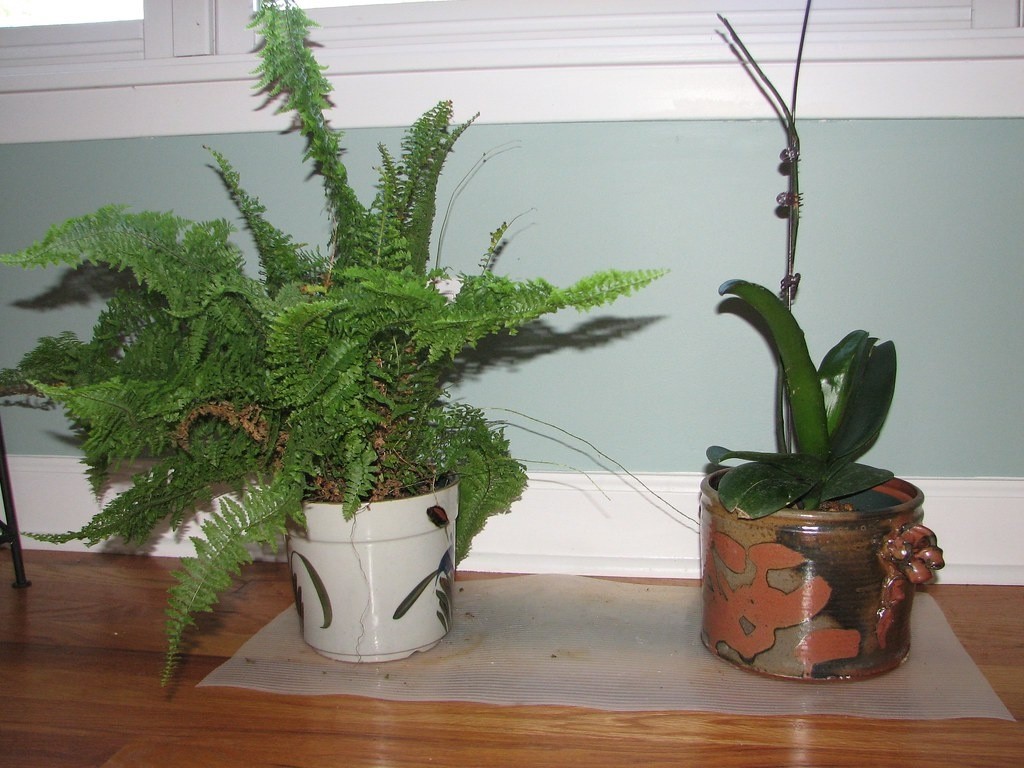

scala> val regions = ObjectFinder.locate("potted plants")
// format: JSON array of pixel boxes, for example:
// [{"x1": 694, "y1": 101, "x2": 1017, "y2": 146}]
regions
[
  {"x1": 0, "y1": 0, "x2": 669, "y2": 685},
  {"x1": 698, "y1": 0, "x2": 947, "y2": 682}
]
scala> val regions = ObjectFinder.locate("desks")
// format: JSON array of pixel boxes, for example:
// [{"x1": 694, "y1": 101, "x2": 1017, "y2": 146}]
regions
[{"x1": 0, "y1": 548, "x2": 1024, "y2": 768}]
